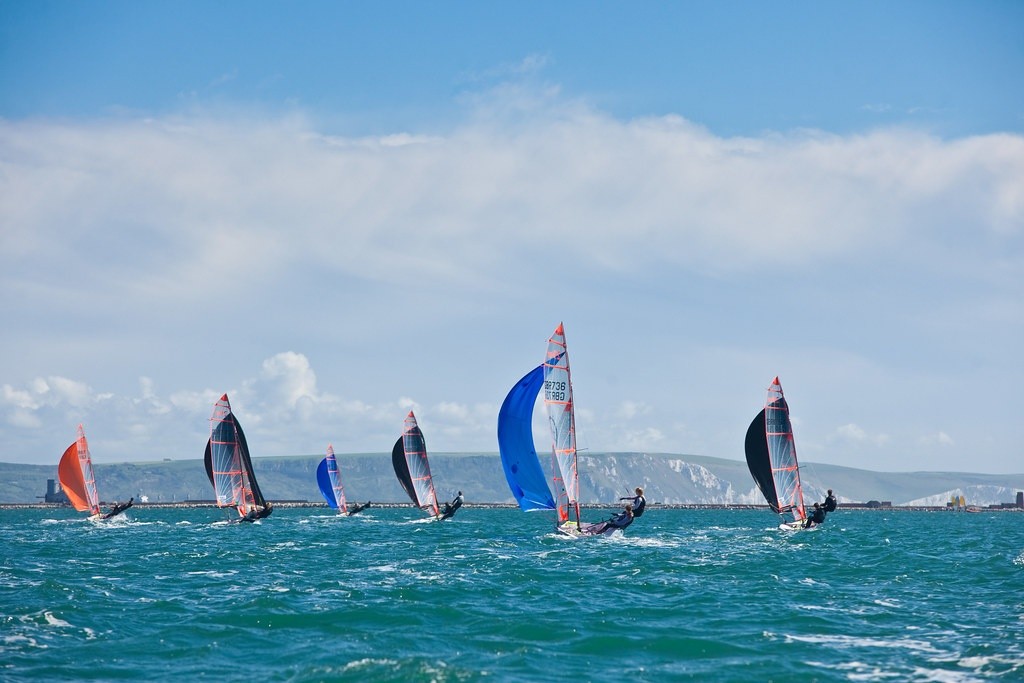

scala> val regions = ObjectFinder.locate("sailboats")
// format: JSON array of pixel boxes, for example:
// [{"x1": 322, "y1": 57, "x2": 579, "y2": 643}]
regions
[
  {"x1": 57, "y1": 436, "x2": 114, "y2": 523},
  {"x1": 391, "y1": 411, "x2": 451, "y2": 524},
  {"x1": 314, "y1": 444, "x2": 367, "y2": 518},
  {"x1": 744, "y1": 376, "x2": 819, "y2": 533},
  {"x1": 203, "y1": 392, "x2": 273, "y2": 526},
  {"x1": 495, "y1": 321, "x2": 625, "y2": 543}
]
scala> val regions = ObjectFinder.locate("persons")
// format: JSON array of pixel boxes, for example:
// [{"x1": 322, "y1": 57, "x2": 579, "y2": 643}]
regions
[
  {"x1": 441, "y1": 502, "x2": 454, "y2": 520},
  {"x1": 239, "y1": 506, "x2": 257, "y2": 523},
  {"x1": 451, "y1": 491, "x2": 464, "y2": 512},
  {"x1": 103, "y1": 498, "x2": 134, "y2": 519},
  {"x1": 257, "y1": 502, "x2": 273, "y2": 519},
  {"x1": 618, "y1": 488, "x2": 646, "y2": 521},
  {"x1": 596, "y1": 505, "x2": 634, "y2": 535},
  {"x1": 349, "y1": 501, "x2": 370, "y2": 516},
  {"x1": 806, "y1": 502, "x2": 825, "y2": 529},
  {"x1": 821, "y1": 490, "x2": 837, "y2": 515}
]
